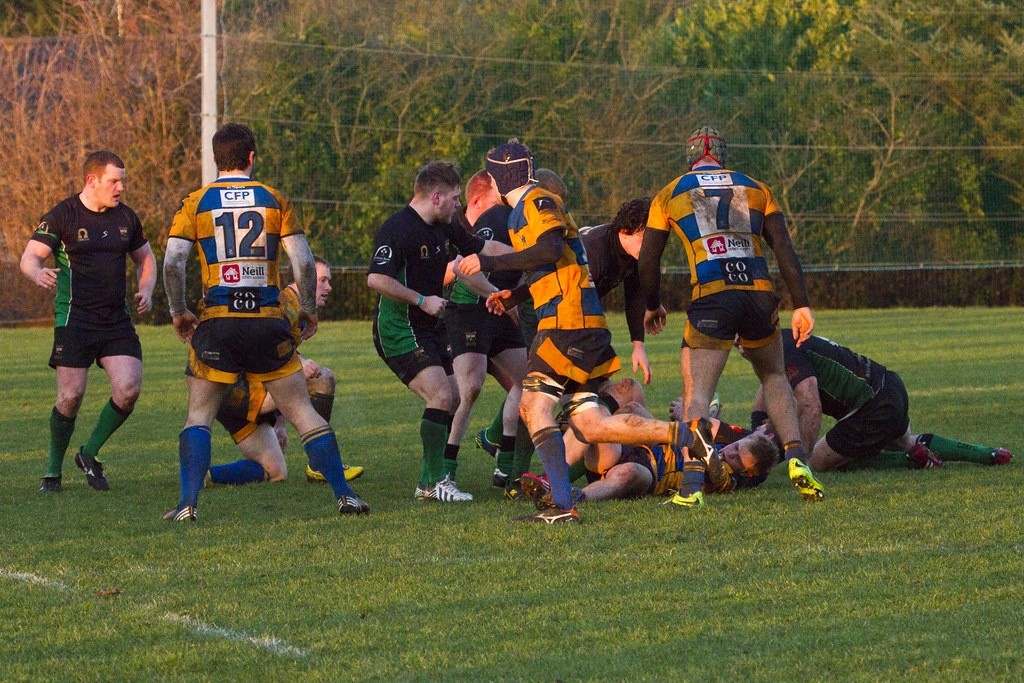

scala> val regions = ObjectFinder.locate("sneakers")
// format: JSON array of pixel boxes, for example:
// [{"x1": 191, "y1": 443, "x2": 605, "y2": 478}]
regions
[
  {"x1": 425, "y1": 473, "x2": 473, "y2": 501},
  {"x1": 339, "y1": 496, "x2": 369, "y2": 513},
  {"x1": 75, "y1": 453, "x2": 110, "y2": 491},
  {"x1": 520, "y1": 472, "x2": 551, "y2": 500},
  {"x1": 40, "y1": 476, "x2": 62, "y2": 492},
  {"x1": 518, "y1": 508, "x2": 582, "y2": 525},
  {"x1": 306, "y1": 465, "x2": 363, "y2": 483},
  {"x1": 503, "y1": 478, "x2": 533, "y2": 502},
  {"x1": 492, "y1": 468, "x2": 509, "y2": 489},
  {"x1": 906, "y1": 444, "x2": 941, "y2": 469},
  {"x1": 789, "y1": 458, "x2": 824, "y2": 501},
  {"x1": 662, "y1": 491, "x2": 704, "y2": 507},
  {"x1": 475, "y1": 428, "x2": 500, "y2": 458},
  {"x1": 163, "y1": 506, "x2": 197, "y2": 521},
  {"x1": 685, "y1": 416, "x2": 724, "y2": 483},
  {"x1": 993, "y1": 447, "x2": 1010, "y2": 465},
  {"x1": 414, "y1": 486, "x2": 424, "y2": 499},
  {"x1": 535, "y1": 487, "x2": 586, "y2": 511}
]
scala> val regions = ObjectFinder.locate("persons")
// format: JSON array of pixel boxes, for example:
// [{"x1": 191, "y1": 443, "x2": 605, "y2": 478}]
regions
[
  {"x1": 441, "y1": 166, "x2": 569, "y2": 489},
  {"x1": 202, "y1": 255, "x2": 363, "y2": 490},
  {"x1": 734, "y1": 325, "x2": 1015, "y2": 470},
  {"x1": 367, "y1": 162, "x2": 515, "y2": 501},
  {"x1": 459, "y1": 142, "x2": 621, "y2": 524},
  {"x1": 163, "y1": 123, "x2": 372, "y2": 521},
  {"x1": 578, "y1": 196, "x2": 654, "y2": 387},
  {"x1": 636, "y1": 125, "x2": 825, "y2": 509},
  {"x1": 522, "y1": 379, "x2": 784, "y2": 511},
  {"x1": 20, "y1": 150, "x2": 157, "y2": 494}
]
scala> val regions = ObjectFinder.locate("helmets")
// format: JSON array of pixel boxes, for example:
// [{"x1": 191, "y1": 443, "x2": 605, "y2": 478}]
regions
[
  {"x1": 686, "y1": 128, "x2": 725, "y2": 166},
  {"x1": 485, "y1": 143, "x2": 533, "y2": 195}
]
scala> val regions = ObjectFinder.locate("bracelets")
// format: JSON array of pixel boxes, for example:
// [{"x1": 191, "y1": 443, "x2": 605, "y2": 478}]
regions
[{"x1": 416, "y1": 294, "x2": 424, "y2": 306}]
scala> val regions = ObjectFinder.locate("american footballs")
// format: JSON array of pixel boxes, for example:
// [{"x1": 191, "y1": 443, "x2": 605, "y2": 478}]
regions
[{"x1": 708, "y1": 391, "x2": 720, "y2": 418}]
